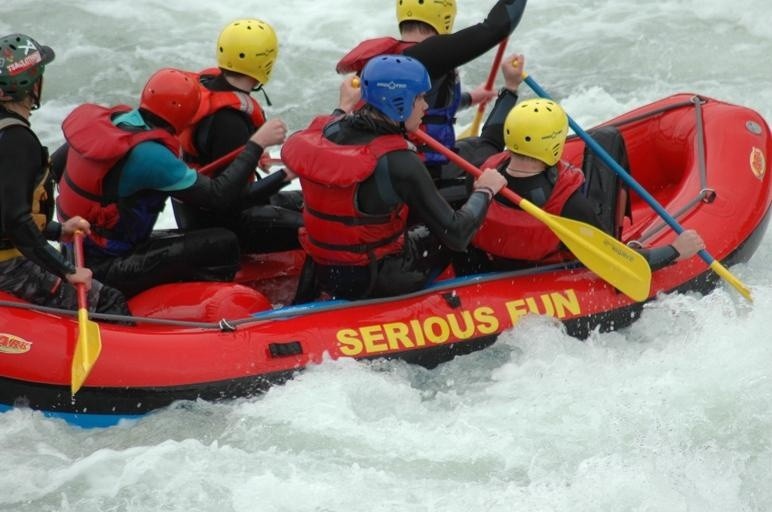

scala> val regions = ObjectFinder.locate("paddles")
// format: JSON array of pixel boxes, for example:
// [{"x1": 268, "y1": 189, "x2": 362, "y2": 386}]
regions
[
  {"x1": 519, "y1": 68, "x2": 754, "y2": 317},
  {"x1": 69, "y1": 231, "x2": 103, "y2": 397},
  {"x1": 415, "y1": 128, "x2": 651, "y2": 303}
]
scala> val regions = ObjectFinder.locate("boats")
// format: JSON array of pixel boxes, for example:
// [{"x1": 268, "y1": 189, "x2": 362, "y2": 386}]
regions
[{"x1": 0, "y1": 94, "x2": 768, "y2": 430}]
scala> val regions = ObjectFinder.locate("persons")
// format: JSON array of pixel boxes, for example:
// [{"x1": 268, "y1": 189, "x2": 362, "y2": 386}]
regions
[
  {"x1": 280, "y1": 55, "x2": 508, "y2": 300},
  {"x1": 1, "y1": 33, "x2": 138, "y2": 326},
  {"x1": 49, "y1": 68, "x2": 287, "y2": 297},
  {"x1": 455, "y1": 54, "x2": 707, "y2": 277},
  {"x1": 336, "y1": 0, "x2": 527, "y2": 181},
  {"x1": 173, "y1": 19, "x2": 304, "y2": 254}
]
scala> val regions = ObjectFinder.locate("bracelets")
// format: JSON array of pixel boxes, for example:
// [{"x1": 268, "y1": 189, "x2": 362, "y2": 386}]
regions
[{"x1": 477, "y1": 187, "x2": 494, "y2": 199}]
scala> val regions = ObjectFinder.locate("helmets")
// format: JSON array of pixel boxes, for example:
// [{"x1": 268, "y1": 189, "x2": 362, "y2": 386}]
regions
[
  {"x1": 217, "y1": 19, "x2": 279, "y2": 85},
  {"x1": 359, "y1": 54, "x2": 432, "y2": 123},
  {"x1": 503, "y1": 99, "x2": 569, "y2": 167},
  {"x1": 396, "y1": 0, "x2": 456, "y2": 35},
  {"x1": 0, "y1": 33, "x2": 56, "y2": 93},
  {"x1": 140, "y1": 68, "x2": 202, "y2": 138}
]
